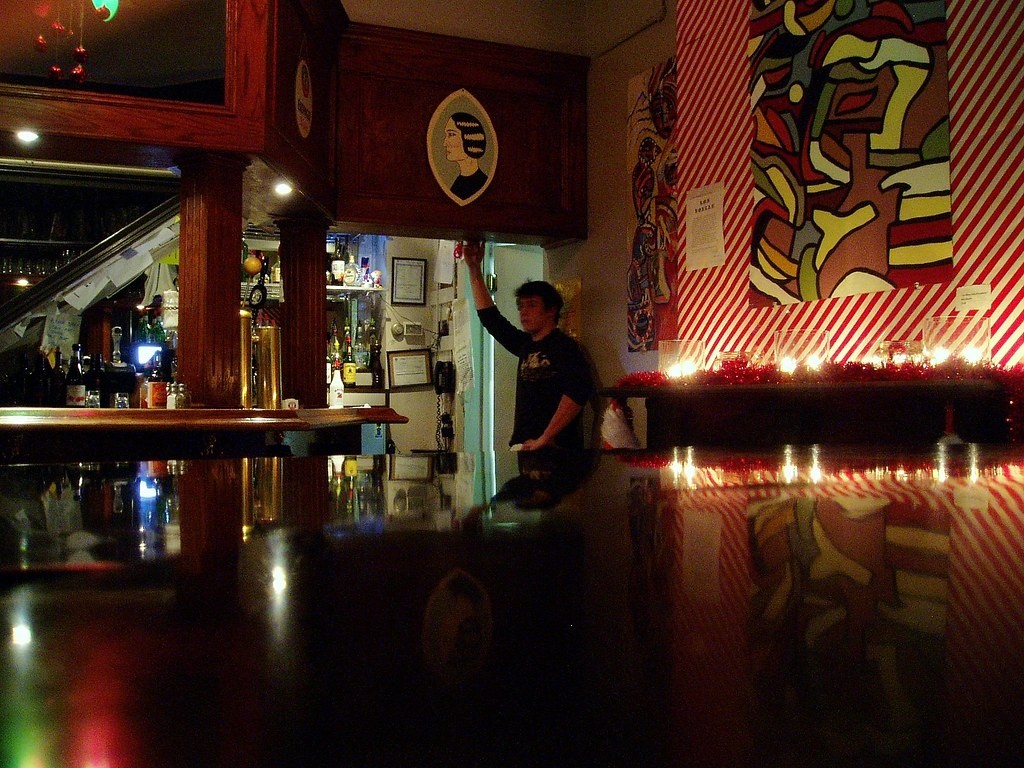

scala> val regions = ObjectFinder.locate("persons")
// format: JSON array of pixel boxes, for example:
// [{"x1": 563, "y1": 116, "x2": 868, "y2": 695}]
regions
[{"x1": 464, "y1": 240, "x2": 599, "y2": 513}]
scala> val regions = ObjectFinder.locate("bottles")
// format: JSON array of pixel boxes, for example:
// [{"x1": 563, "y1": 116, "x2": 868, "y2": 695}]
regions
[
  {"x1": 329, "y1": 470, "x2": 376, "y2": 524},
  {"x1": 167, "y1": 384, "x2": 177, "y2": 409},
  {"x1": 53, "y1": 352, "x2": 66, "y2": 407},
  {"x1": 326, "y1": 340, "x2": 333, "y2": 384},
  {"x1": 66, "y1": 344, "x2": 85, "y2": 407},
  {"x1": 20, "y1": 353, "x2": 51, "y2": 407},
  {"x1": 85, "y1": 354, "x2": 103, "y2": 408},
  {"x1": 330, "y1": 369, "x2": 345, "y2": 409},
  {"x1": 343, "y1": 343, "x2": 356, "y2": 388},
  {"x1": 175, "y1": 384, "x2": 191, "y2": 410}
]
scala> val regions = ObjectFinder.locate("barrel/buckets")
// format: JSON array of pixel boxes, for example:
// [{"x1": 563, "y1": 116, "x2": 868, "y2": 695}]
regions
[{"x1": 147, "y1": 382, "x2": 167, "y2": 409}]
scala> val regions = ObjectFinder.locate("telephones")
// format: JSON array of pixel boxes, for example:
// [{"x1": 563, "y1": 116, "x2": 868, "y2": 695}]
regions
[{"x1": 434, "y1": 361, "x2": 453, "y2": 395}]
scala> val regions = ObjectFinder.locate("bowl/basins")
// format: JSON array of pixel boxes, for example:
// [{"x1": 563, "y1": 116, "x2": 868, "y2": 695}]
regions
[
  {"x1": 657, "y1": 340, "x2": 708, "y2": 380},
  {"x1": 923, "y1": 316, "x2": 992, "y2": 364},
  {"x1": 771, "y1": 329, "x2": 831, "y2": 372}
]
